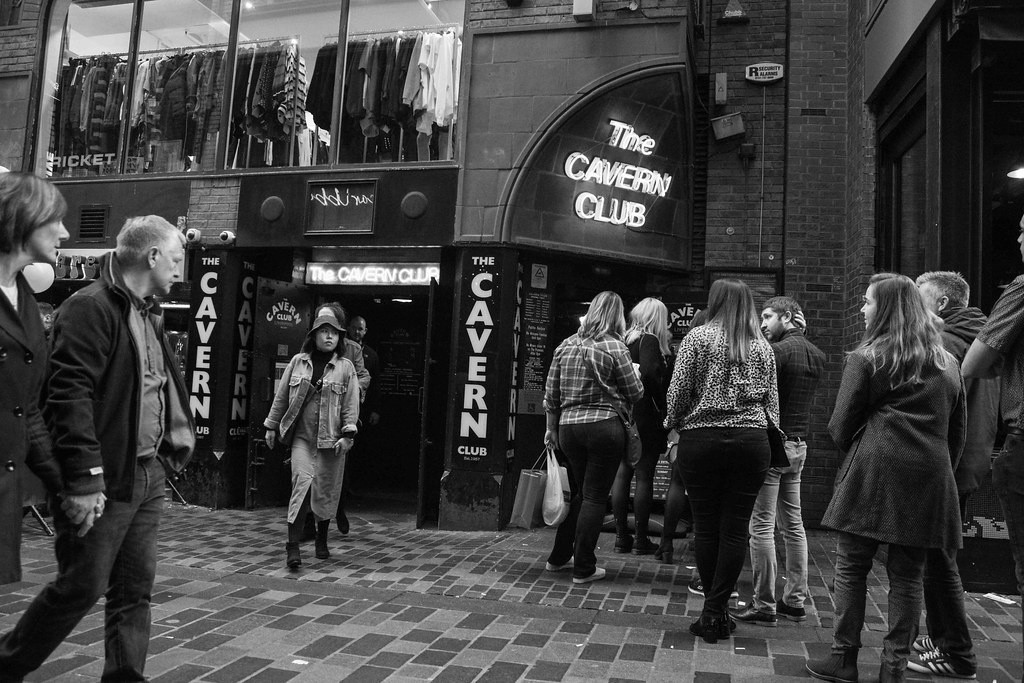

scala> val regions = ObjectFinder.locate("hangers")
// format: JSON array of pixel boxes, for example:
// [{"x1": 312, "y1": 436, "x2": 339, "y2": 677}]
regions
[{"x1": 63, "y1": 22, "x2": 463, "y2": 69}]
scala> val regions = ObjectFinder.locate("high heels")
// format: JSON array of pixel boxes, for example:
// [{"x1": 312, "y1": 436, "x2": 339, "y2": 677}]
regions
[
  {"x1": 688, "y1": 615, "x2": 719, "y2": 643},
  {"x1": 719, "y1": 610, "x2": 732, "y2": 639},
  {"x1": 654, "y1": 544, "x2": 674, "y2": 563}
]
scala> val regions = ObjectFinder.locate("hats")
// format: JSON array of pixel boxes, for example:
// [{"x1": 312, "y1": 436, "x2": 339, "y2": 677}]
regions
[{"x1": 306, "y1": 307, "x2": 347, "y2": 339}]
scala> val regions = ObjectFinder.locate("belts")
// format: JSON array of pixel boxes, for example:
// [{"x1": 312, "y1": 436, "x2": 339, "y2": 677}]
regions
[{"x1": 785, "y1": 436, "x2": 807, "y2": 442}]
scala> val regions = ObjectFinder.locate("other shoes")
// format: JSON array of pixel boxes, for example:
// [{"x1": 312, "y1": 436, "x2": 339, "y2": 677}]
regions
[
  {"x1": 879, "y1": 665, "x2": 907, "y2": 683},
  {"x1": 688, "y1": 577, "x2": 739, "y2": 598},
  {"x1": 806, "y1": 648, "x2": 858, "y2": 683},
  {"x1": 281, "y1": 505, "x2": 350, "y2": 568},
  {"x1": 544, "y1": 555, "x2": 575, "y2": 571},
  {"x1": 573, "y1": 566, "x2": 606, "y2": 583}
]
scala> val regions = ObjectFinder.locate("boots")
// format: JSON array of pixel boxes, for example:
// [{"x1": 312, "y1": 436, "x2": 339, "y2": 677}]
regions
[
  {"x1": 612, "y1": 511, "x2": 635, "y2": 552},
  {"x1": 632, "y1": 514, "x2": 659, "y2": 554}
]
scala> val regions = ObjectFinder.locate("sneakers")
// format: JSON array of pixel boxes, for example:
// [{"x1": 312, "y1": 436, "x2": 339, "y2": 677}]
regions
[
  {"x1": 912, "y1": 635, "x2": 936, "y2": 651},
  {"x1": 907, "y1": 647, "x2": 977, "y2": 679}
]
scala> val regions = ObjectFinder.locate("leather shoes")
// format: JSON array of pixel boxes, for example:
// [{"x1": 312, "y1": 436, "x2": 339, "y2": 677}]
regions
[
  {"x1": 729, "y1": 603, "x2": 778, "y2": 627},
  {"x1": 776, "y1": 598, "x2": 807, "y2": 622}
]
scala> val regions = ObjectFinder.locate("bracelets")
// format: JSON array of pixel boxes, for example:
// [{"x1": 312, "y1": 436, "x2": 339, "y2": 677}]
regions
[{"x1": 547, "y1": 423, "x2": 558, "y2": 431}]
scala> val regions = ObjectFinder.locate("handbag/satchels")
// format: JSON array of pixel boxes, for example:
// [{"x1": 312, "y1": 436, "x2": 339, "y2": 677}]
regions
[
  {"x1": 509, "y1": 446, "x2": 546, "y2": 529},
  {"x1": 542, "y1": 444, "x2": 570, "y2": 527},
  {"x1": 624, "y1": 419, "x2": 643, "y2": 468},
  {"x1": 764, "y1": 425, "x2": 790, "y2": 469},
  {"x1": 643, "y1": 407, "x2": 671, "y2": 432}
]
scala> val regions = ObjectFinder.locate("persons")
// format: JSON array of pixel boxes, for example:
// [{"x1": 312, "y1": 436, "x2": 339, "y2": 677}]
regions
[
  {"x1": 727, "y1": 294, "x2": 826, "y2": 628},
  {"x1": 1, "y1": 171, "x2": 107, "y2": 584},
  {"x1": 665, "y1": 278, "x2": 791, "y2": 643},
  {"x1": 37, "y1": 300, "x2": 54, "y2": 336},
  {"x1": 612, "y1": 298, "x2": 711, "y2": 563},
  {"x1": 806, "y1": 272, "x2": 967, "y2": 682},
  {"x1": 263, "y1": 303, "x2": 380, "y2": 567},
  {"x1": 0, "y1": 215, "x2": 199, "y2": 683},
  {"x1": 907, "y1": 212, "x2": 1024, "y2": 678},
  {"x1": 542, "y1": 291, "x2": 645, "y2": 583}
]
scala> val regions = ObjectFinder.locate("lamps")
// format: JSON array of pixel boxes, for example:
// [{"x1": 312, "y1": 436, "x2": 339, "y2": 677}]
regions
[{"x1": 1007, "y1": 158, "x2": 1024, "y2": 180}]
